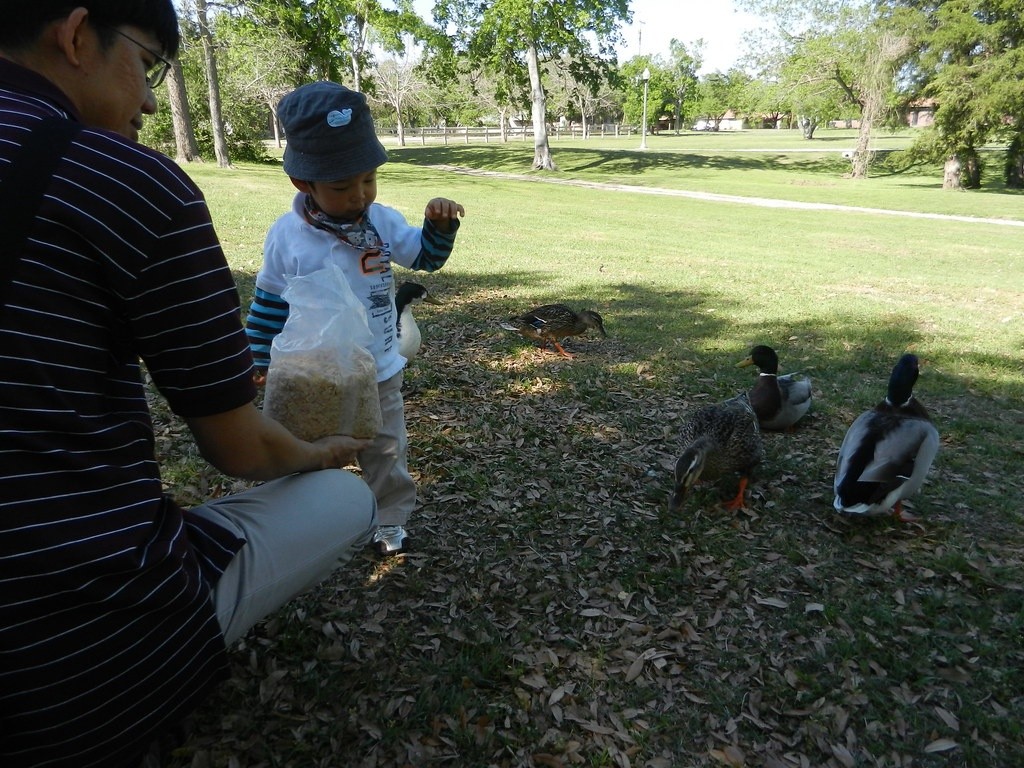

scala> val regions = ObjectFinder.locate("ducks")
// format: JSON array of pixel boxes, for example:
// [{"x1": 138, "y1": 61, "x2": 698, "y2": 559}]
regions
[
  {"x1": 733, "y1": 345, "x2": 813, "y2": 435},
  {"x1": 498, "y1": 304, "x2": 607, "y2": 359},
  {"x1": 834, "y1": 354, "x2": 940, "y2": 526},
  {"x1": 395, "y1": 281, "x2": 443, "y2": 365},
  {"x1": 669, "y1": 391, "x2": 763, "y2": 514}
]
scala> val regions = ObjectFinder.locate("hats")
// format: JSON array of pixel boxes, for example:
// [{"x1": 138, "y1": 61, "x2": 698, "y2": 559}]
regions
[{"x1": 276, "y1": 79, "x2": 388, "y2": 183}]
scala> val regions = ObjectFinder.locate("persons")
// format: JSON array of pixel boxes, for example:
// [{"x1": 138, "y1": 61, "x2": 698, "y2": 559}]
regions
[
  {"x1": 242, "y1": 80, "x2": 466, "y2": 559},
  {"x1": 0, "y1": 0, "x2": 380, "y2": 768}
]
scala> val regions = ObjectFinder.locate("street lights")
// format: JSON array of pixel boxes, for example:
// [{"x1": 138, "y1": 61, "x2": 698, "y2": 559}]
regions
[{"x1": 640, "y1": 67, "x2": 651, "y2": 148}]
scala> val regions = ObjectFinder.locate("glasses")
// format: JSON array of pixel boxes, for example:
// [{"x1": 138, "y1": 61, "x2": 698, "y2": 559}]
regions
[{"x1": 101, "y1": 23, "x2": 173, "y2": 91}]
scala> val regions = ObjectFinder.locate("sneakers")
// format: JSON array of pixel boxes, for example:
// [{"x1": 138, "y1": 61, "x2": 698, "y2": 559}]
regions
[{"x1": 373, "y1": 524, "x2": 411, "y2": 556}]
